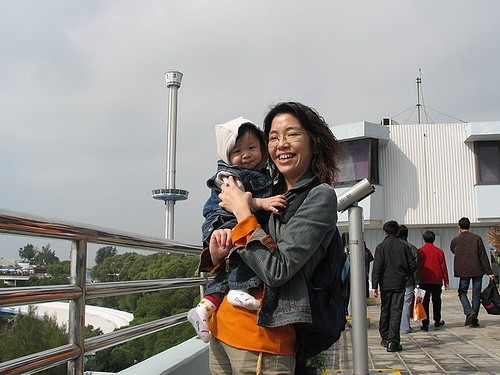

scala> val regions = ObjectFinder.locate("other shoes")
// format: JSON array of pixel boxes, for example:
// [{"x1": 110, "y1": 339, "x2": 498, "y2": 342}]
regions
[
  {"x1": 472, "y1": 318, "x2": 479, "y2": 327},
  {"x1": 421, "y1": 326, "x2": 428, "y2": 331},
  {"x1": 381, "y1": 340, "x2": 388, "y2": 347},
  {"x1": 387, "y1": 342, "x2": 402, "y2": 352},
  {"x1": 434, "y1": 321, "x2": 444, "y2": 327},
  {"x1": 465, "y1": 312, "x2": 476, "y2": 326},
  {"x1": 401, "y1": 327, "x2": 416, "y2": 334}
]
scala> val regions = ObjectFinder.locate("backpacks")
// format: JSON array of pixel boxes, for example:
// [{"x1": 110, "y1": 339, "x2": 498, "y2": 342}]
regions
[{"x1": 283, "y1": 179, "x2": 349, "y2": 363}]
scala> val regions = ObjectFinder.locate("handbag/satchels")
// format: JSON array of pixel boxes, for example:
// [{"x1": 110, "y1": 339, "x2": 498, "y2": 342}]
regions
[
  {"x1": 414, "y1": 288, "x2": 428, "y2": 322},
  {"x1": 479, "y1": 280, "x2": 500, "y2": 315}
]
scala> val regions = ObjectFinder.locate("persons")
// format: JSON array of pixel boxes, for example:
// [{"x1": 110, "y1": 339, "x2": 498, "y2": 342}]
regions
[
  {"x1": 450, "y1": 217, "x2": 496, "y2": 328},
  {"x1": 187, "y1": 117, "x2": 288, "y2": 344},
  {"x1": 372, "y1": 220, "x2": 416, "y2": 352},
  {"x1": 418, "y1": 231, "x2": 449, "y2": 331},
  {"x1": 196, "y1": 101, "x2": 342, "y2": 375},
  {"x1": 340, "y1": 237, "x2": 350, "y2": 330},
  {"x1": 397, "y1": 225, "x2": 419, "y2": 334},
  {"x1": 364, "y1": 241, "x2": 373, "y2": 299}
]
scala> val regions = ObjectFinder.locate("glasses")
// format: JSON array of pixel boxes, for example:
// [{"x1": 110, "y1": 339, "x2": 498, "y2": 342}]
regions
[{"x1": 265, "y1": 132, "x2": 308, "y2": 146}]
